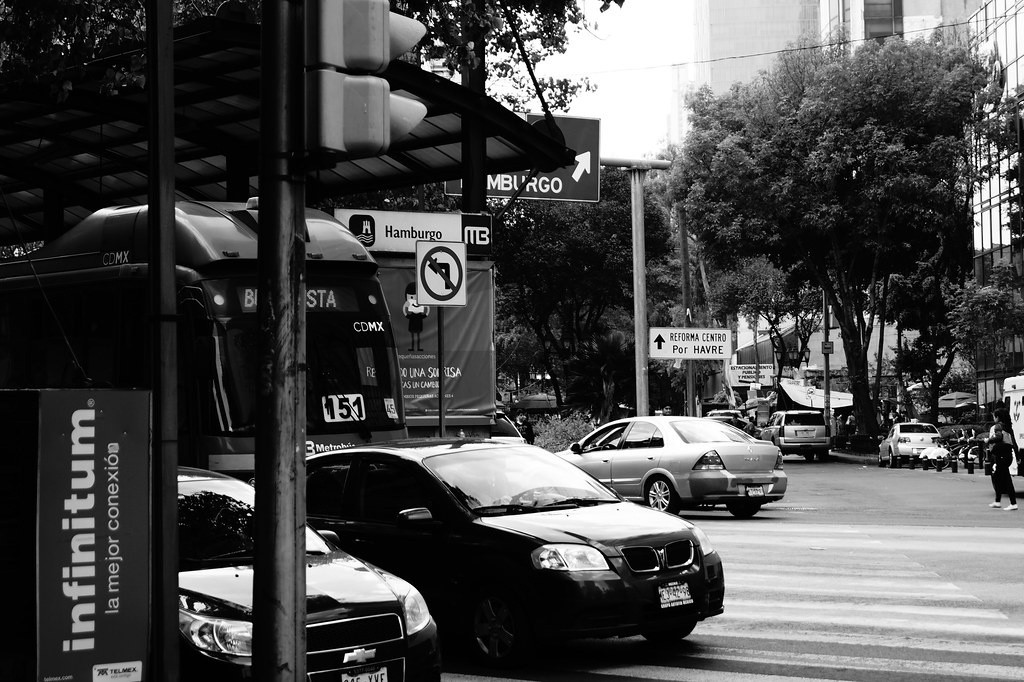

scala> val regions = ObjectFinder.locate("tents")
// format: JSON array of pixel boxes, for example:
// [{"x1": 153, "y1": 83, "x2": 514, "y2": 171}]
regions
[{"x1": 777, "y1": 382, "x2": 853, "y2": 410}]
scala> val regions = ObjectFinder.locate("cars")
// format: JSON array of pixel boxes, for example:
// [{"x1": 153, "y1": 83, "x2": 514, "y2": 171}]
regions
[
  {"x1": 172, "y1": 464, "x2": 444, "y2": 682},
  {"x1": 551, "y1": 416, "x2": 787, "y2": 520},
  {"x1": 303, "y1": 435, "x2": 725, "y2": 679},
  {"x1": 705, "y1": 416, "x2": 762, "y2": 441},
  {"x1": 877, "y1": 421, "x2": 945, "y2": 469}
]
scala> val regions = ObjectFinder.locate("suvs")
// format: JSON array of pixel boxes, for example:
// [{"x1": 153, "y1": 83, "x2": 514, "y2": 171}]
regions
[
  {"x1": 706, "y1": 408, "x2": 744, "y2": 418},
  {"x1": 409, "y1": 409, "x2": 527, "y2": 442},
  {"x1": 760, "y1": 411, "x2": 831, "y2": 464}
]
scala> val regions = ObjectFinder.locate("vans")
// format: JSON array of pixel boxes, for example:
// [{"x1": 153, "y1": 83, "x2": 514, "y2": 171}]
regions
[{"x1": 1002, "y1": 371, "x2": 1024, "y2": 476}]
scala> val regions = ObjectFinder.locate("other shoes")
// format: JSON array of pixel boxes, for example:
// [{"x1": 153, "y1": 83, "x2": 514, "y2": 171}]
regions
[
  {"x1": 989, "y1": 502, "x2": 1001, "y2": 508},
  {"x1": 1003, "y1": 504, "x2": 1018, "y2": 510}
]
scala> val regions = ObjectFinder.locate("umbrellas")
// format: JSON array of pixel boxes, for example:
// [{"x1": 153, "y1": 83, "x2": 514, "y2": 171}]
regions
[{"x1": 939, "y1": 391, "x2": 977, "y2": 416}]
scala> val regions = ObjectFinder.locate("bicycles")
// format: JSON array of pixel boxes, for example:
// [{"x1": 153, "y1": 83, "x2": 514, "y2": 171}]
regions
[{"x1": 928, "y1": 428, "x2": 988, "y2": 470}]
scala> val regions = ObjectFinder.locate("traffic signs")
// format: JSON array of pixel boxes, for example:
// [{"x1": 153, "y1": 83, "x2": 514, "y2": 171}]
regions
[
  {"x1": 445, "y1": 112, "x2": 601, "y2": 203},
  {"x1": 648, "y1": 326, "x2": 731, "y2": 360}
]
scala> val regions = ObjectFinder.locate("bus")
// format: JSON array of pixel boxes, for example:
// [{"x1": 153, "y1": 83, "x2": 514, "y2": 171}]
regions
[{"x1": 0, "y1": 201, "x2": 413, "y2": 484}]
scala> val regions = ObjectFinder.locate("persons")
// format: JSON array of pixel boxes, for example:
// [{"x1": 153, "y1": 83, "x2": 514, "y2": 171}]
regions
[
  {"x1": 743, "y1": 416, "x2": 761, "y2": 437},
  {"x1": 983, "y1": 410, "x2": 1019, "y2": 511},
  {"x1": 662, "y1": 403, "x2": 672, "y2": 416},
  {"x1": 512, "y1": 411, "x2": 535, "y2": 445}
]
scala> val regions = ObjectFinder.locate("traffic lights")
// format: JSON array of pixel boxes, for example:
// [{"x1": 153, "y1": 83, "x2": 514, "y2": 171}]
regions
[{"x1": 306, "y1": 0, "x2": 427, "y2": 161}]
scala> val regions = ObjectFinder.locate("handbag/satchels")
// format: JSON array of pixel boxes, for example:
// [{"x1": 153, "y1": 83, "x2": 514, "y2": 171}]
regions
[{"x1": 988, "y1": 424, "x2": 1013, "y2": 452}]
